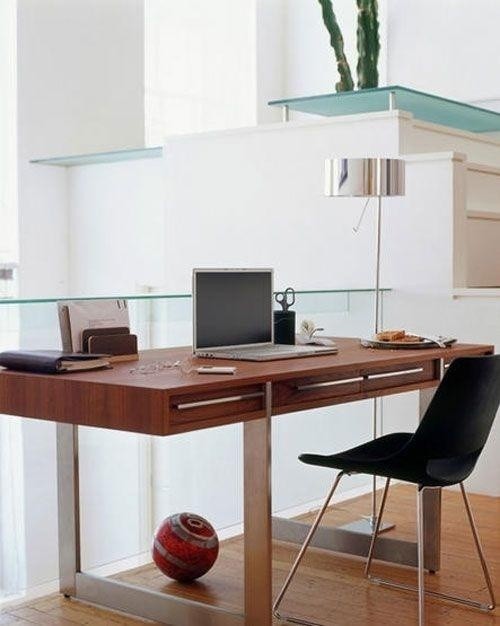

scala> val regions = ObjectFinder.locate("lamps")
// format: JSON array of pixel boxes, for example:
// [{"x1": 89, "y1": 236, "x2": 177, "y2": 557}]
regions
[{"x1": 322, "y1": 156, "x2": 407, "y2": 537}]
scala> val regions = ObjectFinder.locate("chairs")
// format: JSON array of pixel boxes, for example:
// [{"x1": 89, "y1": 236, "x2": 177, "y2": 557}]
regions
[{"x1": 272, "y1": 353, "x2": 500, "y2": 626}]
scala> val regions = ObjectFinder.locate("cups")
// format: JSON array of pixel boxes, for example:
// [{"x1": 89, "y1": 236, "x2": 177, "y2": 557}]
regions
[{"x1": 273, "y1": 309, "x2": 295, "y2": 345}]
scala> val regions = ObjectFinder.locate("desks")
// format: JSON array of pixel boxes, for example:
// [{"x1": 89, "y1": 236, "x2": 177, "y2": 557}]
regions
[{"x1": 1, "y1": 335, "x2": 495, "y2": 625}]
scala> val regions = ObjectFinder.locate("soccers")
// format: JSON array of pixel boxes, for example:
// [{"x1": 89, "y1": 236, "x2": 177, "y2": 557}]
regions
[{"x1": 152, "y1": 513, "x2": 219, "y2": 583}]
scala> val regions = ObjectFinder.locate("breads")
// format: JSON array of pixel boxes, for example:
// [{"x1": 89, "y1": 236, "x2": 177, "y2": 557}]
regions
[{"x1": 376, "y1": 330, "x2": 422, "y2": 343}]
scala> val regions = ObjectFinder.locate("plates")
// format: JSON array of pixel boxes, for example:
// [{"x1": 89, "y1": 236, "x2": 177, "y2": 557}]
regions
[{"x1": 361, "y1": 332, "x2": 457, "y2": 349}]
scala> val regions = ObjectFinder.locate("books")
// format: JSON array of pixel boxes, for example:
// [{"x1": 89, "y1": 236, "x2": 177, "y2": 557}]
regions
[{"x1": 0, "y1": 349, "x2": 114, "y2": 374}]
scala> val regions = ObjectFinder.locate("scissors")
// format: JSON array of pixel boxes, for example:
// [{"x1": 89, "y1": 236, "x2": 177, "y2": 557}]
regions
[{"x1": 275, "y1": 288, "x2": 295, "y2": 311}]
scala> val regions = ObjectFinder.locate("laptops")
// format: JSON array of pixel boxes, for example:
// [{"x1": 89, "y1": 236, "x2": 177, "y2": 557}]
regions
[{"x1": 190, "y1": 266, "x2": 339, "y2": 361}]
